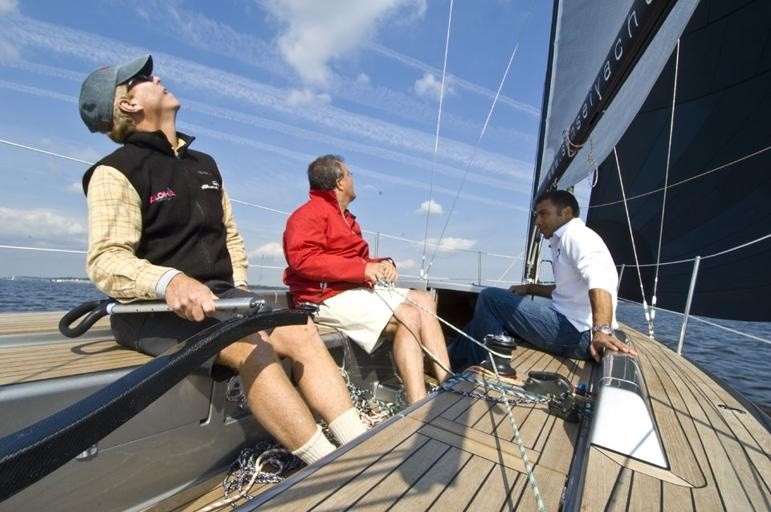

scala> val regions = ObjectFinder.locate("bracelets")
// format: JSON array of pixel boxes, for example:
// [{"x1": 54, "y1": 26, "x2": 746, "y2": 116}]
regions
[{"x1": 590, "y1": 324, "x2": 613, "y2": 335}]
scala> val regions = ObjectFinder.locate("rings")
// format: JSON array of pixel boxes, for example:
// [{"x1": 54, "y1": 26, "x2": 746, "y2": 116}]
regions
[{"x1": 380, "y1": 262, "x2": 385, "y2": 267}]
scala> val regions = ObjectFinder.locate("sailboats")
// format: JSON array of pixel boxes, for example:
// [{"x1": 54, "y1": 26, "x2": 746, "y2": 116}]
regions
[{"x1": 0, "y1": 0, "x2": 771, "y2": 511}]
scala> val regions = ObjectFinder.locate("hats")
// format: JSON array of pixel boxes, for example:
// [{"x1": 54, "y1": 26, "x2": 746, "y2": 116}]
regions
[{"x1": 78, "y1": 53, "x2": 152, "y2": 133}]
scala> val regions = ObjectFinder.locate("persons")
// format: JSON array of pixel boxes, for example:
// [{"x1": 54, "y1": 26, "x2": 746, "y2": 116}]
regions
[
  {"x1": 72, "y1": 50, "x2": 370, "y2": 468},
  {"x1": 441, "y1": 190, "x2": 642, "y2": 366},
  {"x1": 279, "y1": 152, "x2": 455, "y2": 406}
]
